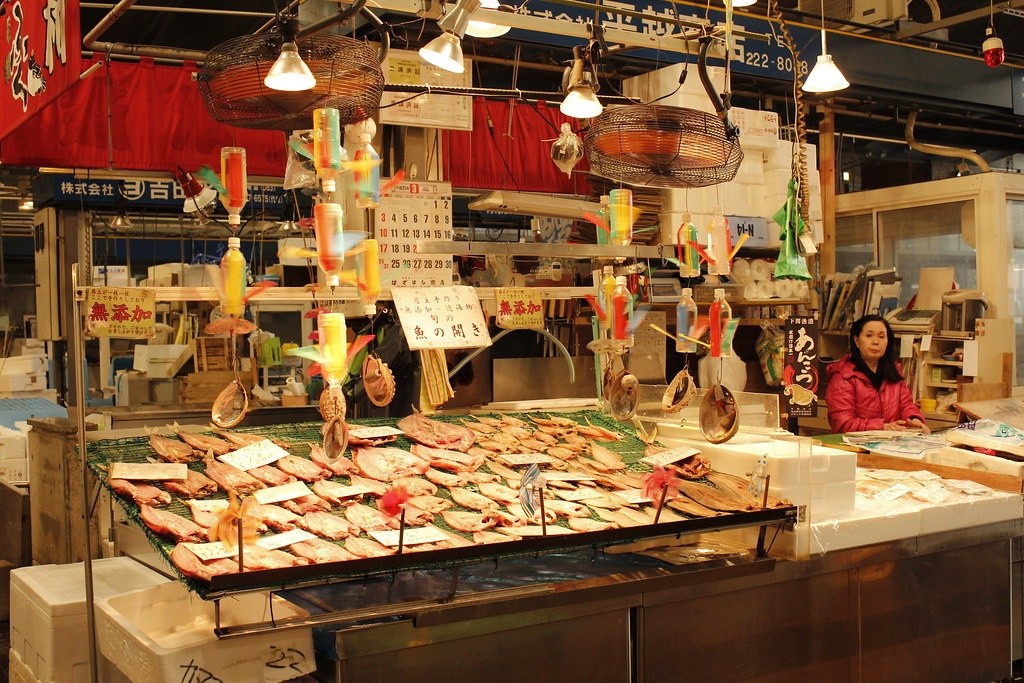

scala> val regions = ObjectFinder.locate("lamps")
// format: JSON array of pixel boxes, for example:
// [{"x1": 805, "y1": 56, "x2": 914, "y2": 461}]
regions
[
  {"x1": 418, "y1": 0, "x2": 513, "y2": 72},
  {"x1": 263, "y1": 0, "x2": 317, "y2": 92},
  {"x1": 559, "y1": 45, "x2": 603, "y2": 118},
  {"x1": 801, "y1": 0, "x2": 850, "y2": 93}
]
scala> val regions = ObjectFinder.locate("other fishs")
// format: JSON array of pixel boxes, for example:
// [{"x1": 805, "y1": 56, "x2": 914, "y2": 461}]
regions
[{"x1": 97, "y1": 403, "x2": 795, "y2": 581}]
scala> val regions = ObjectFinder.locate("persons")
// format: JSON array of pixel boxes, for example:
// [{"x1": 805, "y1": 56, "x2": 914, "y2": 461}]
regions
[{"x1": 825, "y1": 315, "x2": 931, "y2": 435}]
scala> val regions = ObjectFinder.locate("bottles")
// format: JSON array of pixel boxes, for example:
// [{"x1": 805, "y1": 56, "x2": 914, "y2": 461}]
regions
[
  {"x1": 220, "y1": 236, "x2": 247, "y2": 315},
  {"x1": 677, "y1": 213, "x2": 700, "y2": 278},
  {"x1": 219, "y1": 148, "x2": 247, "y2": 224},
  {"x1": 709, "y1": 289, "x2": 733, "y2": 359},
  {"x1": 598, "y1": 196, "x2": 610, "y2": 246},
  {"x1": 313, "y1": 204, "x2": 345, "y2": 287},
  {"x1": 355, "y1": 132, "x2": 382, "y2": 209},
  {"x1": 598, "y1": 266, "x2": 616, "y2": 330},
  {"x1": 312, "y1": 109, "x2": 341, "y2": 195},
  {"x1": 709, "y1": 206, "x2": 731, "y2": 276},
  {"x1": 612, "y1": 190, "x2": 632, "y2": 263},
  {"x1": 318, "y1": 312, "x2": 348, "y2": 398},
  {"x1": 676, "y1": 287, "x2": 698, "y2": 353},
  {"x1": 609, "y1": 275, "x2": 635, "y2": 348},
  {"x1": 354, "y1": 239, "x2": 381, "y2": 315}
]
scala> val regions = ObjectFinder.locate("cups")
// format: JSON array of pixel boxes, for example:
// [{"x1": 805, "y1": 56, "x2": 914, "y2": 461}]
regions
[
  {"x1": 282, "y1": 395, "x2": 309, "y2": 407},
  {"x1": 915, "y1": 398, "x2": 937, "y2": 411}
]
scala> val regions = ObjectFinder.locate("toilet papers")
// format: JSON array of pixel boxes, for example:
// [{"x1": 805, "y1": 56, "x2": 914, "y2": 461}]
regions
[{"x1": 285, "y1": 377, "x2": 301, "y2": 396}]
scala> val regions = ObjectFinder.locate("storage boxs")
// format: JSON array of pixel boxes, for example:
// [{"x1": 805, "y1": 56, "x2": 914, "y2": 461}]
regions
[
  {"x1": 8, "y1": 558, "x2": 317, "y2": 683},
  {"x1": 622, "y1": 63, "x2": 825, "y2": 246},
  {"x1": 0, "y1": 420, "x2": 33, "y2": 483},
  {"x1": 0, "y1": 338, "x2": 69, "y2": 485},
  {"x1": 650, "y1": 428, "x2": 857, "y2": 522}
]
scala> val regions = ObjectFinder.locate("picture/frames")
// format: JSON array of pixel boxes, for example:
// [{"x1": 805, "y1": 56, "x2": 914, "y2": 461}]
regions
[{"x1": 649, "y1": 278, "x2": 683, "y2": 302}]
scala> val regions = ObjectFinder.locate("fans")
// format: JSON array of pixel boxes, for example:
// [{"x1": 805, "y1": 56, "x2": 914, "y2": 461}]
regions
[
  {"x1": 191, "y1": 31, "x2": 384, "y2": 133},
  {"x1": 583, "y1": 105, "x2": 745, "y2": 189}
]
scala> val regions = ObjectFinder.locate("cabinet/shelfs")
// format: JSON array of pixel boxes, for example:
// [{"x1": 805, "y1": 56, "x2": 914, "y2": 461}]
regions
[
  {"x1": 798, "y1": 316, "x2": 1013, "y2": 436},
  {"x1": 62, "y1": 241, "x2": 809, "y2": 429}
]
